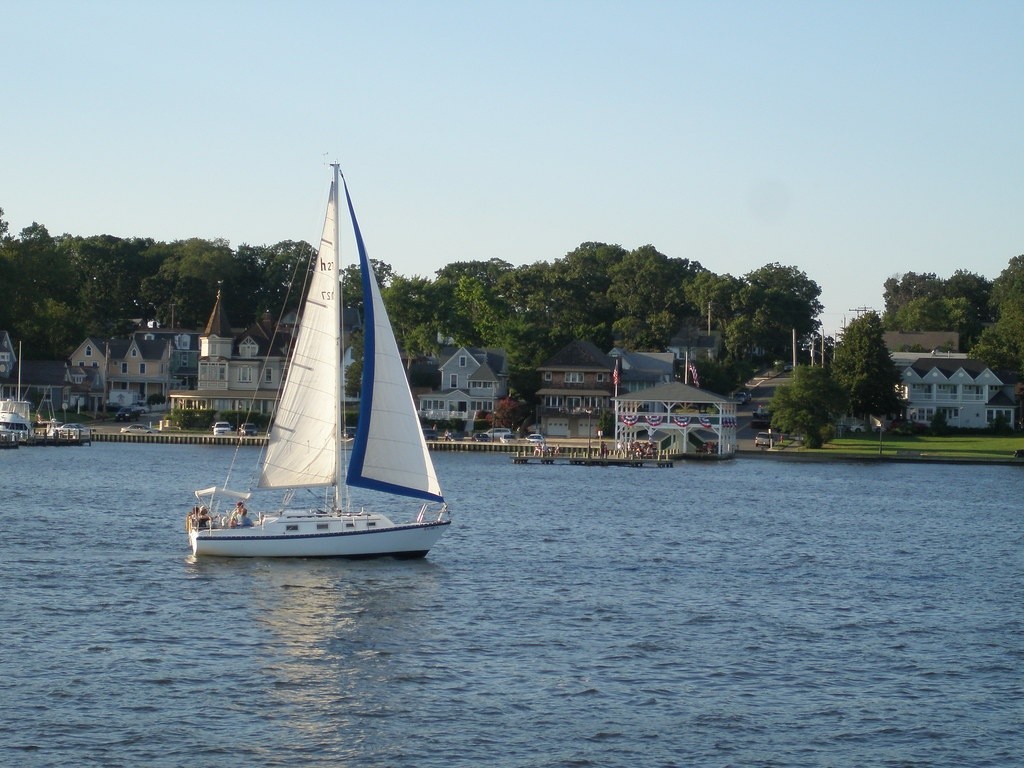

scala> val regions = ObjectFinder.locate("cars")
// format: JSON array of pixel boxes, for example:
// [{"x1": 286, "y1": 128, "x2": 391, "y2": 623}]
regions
[
  {"x1": 121, "y1": 424, "x2": 160, "y2": 434},
  {"x1": 129, "y1": 403, "x2": 151, "y2": 414},
  {"x1": 115, "y1": 407, "x2": 140, "y2": 422},
  {"x1": 423, "y1": 429, "x2": 439, "y2": 441},
  {"x1": 341, "y1": 427, "x2": 356, "y2": 437},
  {"x1": 472, "y1": 434, "x2": 490, "y2": 442},
  {"x1": 785, "y1": 363, "x2": 793, "y2": 371},
  {"x1": 239, "y1": 423, "x2": 259, "y2": 436},
  {"x1": 851, "y1": 423, "x2": 887, "y2": 434},
  {"x1": 55, "y1": 423, "x2": 89, "y2": 433},
  {"x1": 751, "y1": 411, "x2": 771, "y2": 429},
  {"x1": 755, "y1": 432, "x2": 775, "y2": 448},
  {"x1": 499, "y1": 434, "x2": 515, "y2": 443},
  {"x1": 480, "y1": 428, "x2": 511, "y2": 438},
  {"x1": 526, "y1": 434, "x2": 544, "y2": 443},
  {"x1": 212, "y1": 421, "x2": 233, "y2": 435}
]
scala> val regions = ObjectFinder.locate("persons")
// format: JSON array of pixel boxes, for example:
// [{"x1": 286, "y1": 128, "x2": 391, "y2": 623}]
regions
[
  {"x1": 230, "y1": 502, "x2": 254, "y2": 528},
  {"x1": 189, "y1": 507, "x2": 213, "y2": 530},
  {"x1": 617, "y1": 441, "x2": 654, "y2": 459},
  {"x1": 534, "y1": 443, "x2": 541, "y2": 456},
  {"x1": 542, "y1": 442, "x2": 550, "y2": 456},
  {"x1": 599, "y1": 441, "x2": 607, "y2": 459},
  {"x1": 701, "y1": 441, "x2": 718, "y2": 454},
  {"x1": 444, "y1": 429, "x2": 449, "y2": 442},
  {"x1": 555, "y1": 444, "x2": 559, "y2": 454}
]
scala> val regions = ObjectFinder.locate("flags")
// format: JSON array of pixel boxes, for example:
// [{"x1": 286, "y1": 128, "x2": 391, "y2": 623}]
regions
[
  {"x1": 613, "y1": 363, "x2": 620, "y2": 384},
  {"x1": 689, "y1": 358, "x2": 699, "y2": 387}
]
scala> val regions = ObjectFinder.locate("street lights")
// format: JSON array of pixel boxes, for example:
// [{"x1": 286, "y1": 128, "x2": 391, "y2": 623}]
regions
[
  {"x1": 586, "y1": 409, "x2": 591, "y2": 454},
  {"x1": 811, "y1": 349, "x2": 824, "y2": 368},
  {"x1": 802, "y1": 344, "x2": 815, "y2": 367}
]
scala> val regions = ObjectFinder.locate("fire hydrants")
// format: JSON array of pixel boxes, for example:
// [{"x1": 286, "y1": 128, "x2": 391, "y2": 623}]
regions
[{"x1": 781, "y1": 436, "x2": 784, "y2": 443}]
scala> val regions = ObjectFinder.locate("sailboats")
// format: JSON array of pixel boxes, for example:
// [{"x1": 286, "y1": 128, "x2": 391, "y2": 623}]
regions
[{"x1": 188, "y1": 163, "x2": 452, "y2": 562}]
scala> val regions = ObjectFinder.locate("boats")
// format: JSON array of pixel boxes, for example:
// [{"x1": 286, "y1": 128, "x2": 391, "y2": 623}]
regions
[{"x1": 0, "y1": 404, "x2": 32, "y2": 436}]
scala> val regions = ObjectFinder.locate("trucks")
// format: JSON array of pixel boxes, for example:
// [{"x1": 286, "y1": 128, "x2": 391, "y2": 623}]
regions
[{"x1": 734, "y1": 390, "x2": 751, "y2": 405}]
